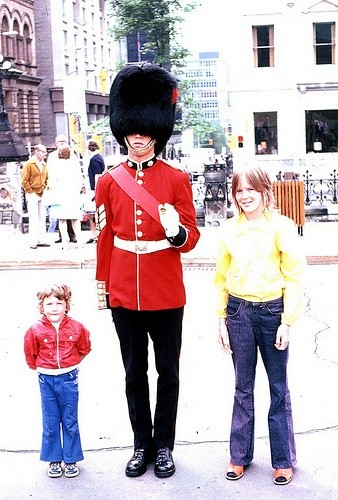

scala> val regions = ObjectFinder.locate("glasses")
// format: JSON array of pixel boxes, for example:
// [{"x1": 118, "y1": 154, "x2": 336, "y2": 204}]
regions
[{"x1": 38, "y1": 150, "x2": 47, "y2": 155}]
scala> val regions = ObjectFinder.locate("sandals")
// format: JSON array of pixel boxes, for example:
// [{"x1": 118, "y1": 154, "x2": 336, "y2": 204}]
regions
[
  {"x1": 273, "y1": 465, "x2": 293, "y2": 484},
  {"x1": 48, "y1": 461, "x2": 62, "y2": 477},
  {"x1": 225, "y1": 462, "x2": 244, "y2": 479},
  {"x1": 64, "y1": 463, "x2": 79, "y2": 478}
]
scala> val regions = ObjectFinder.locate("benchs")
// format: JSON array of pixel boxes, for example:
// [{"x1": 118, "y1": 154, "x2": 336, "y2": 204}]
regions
[{"x1": 305, "y1": 207, "x2": 328, "y2": 220}]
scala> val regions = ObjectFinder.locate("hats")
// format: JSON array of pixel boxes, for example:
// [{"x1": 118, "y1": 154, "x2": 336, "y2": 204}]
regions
[{"x1": 109, "y1": 64, "x2": 178, "y2": 156}]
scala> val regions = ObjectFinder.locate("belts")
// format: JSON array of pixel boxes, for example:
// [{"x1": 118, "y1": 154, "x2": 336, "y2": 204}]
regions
[{"x1": 113, "y1": 235, "x2": 172, "y2": 254}]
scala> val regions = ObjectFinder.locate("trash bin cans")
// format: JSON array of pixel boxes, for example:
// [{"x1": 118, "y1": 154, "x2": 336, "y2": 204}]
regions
[
  {"x1": 204, "y1": 166, "x2": 227, "y2": 227},
  {"x1": 270, "y1": 179, "x2": 304, "y2": 236}
]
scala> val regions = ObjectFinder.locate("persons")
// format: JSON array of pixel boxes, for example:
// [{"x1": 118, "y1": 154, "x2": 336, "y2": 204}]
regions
[
  {"x1": 94, "y1": 64, "x2": 202, "y2": 480},
  {"x1": 212, "y1": 164, "x2": 308, "y2": 486},
  {"x1": 23, "y1": 279, "x2": 92, "y2": 478},
  {"x1": 20, "y1": 133, "x2": 107, "y2": 250}
]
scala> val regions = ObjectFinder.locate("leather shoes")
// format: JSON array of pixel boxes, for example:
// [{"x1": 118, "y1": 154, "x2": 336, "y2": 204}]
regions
[
  {"x1": 126, "y1": 447, "x2": 151, "y2": 476},
  {"x1": 154, "y1": 447, "x2": 176, "y2": 477}
]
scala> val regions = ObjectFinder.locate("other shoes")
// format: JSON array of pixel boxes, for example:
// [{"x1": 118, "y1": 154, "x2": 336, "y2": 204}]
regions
[
  {"x1": 30, "y1": 245, "x2": 37, "y2": 249},
  {"x1": 37, "y1": 243, "x2": 50, "y2": 247},
  {"x1": 86, "y1": 238, "x2": 94, "y2": 243},
  {"x1": 55, "y1": 239, "x2": 63, "y2": 243},
  {"x1": 70, "y1": 239, "x2": 77, "y2": 242}
]
